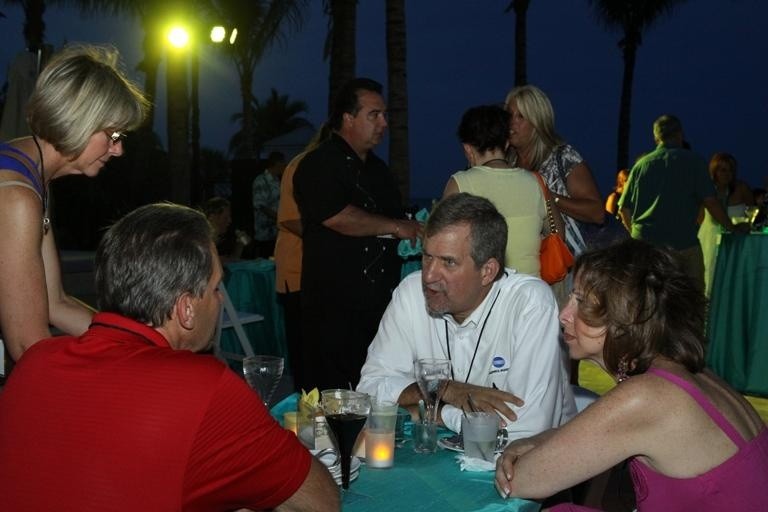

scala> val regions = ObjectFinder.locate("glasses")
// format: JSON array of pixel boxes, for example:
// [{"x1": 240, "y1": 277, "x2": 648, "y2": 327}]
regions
[{"x1": 103, "y1": 128, "x2": 128, "y2": 145}]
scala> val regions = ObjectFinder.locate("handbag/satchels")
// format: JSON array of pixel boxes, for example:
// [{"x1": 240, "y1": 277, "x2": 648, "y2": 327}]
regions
[
  {"x1": 563, "y1": 210, "x2": 632, "y2": 261},
  {"x1": 540, "y1": 233, "x2": 577, "y2": 285}
]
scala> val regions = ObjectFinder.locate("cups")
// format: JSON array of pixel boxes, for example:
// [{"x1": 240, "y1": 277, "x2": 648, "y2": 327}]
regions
[
  {"x1": 462, "y1": 412, "x2": 499, "y2": 463},
  {"x1": 363, "y1": 400, "x2": 397, "y2": 472}
]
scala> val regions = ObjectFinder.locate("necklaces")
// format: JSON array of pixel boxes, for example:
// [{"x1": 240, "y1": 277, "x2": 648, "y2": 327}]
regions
[{"x1": 34, "y1": 133, "x2": 50, "y2": 235}]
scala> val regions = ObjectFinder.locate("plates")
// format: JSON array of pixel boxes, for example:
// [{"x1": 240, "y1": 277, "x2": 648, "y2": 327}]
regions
[{"x1": 278, "y1": 447, "x2": 363, "y2": 483}]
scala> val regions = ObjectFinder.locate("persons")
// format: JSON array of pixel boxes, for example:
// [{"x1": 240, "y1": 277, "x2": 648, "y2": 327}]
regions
[
  {"x1": 2, "y1": 43, "x2": 149, "y2": 364},
  {"x1": 181, "y1": 75, "x2": 761, "y2": 333},
  {"x1": 2, "y1": 198, "x2": 340, "y2": 510},
  {"x1": 349, "y1": 186, "x2": 588, "y2": 476},
  {"x1": 493, "y1": 239, "x2": 765, "y2": 511}
]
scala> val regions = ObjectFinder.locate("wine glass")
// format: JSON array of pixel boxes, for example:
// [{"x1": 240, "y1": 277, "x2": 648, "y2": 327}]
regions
[
  {"x1": 742, "y1": 206, "x2": 759, "y2": 228},
  {"x1": 240, "y1": 358, "x2": 286, "y2": 412},
  {"x1": 320, "y1": 389, "x2": 372, "y2": 499},
  {"x1": 416, "y1": 359, "x2": 452, "y2": 443}
]
